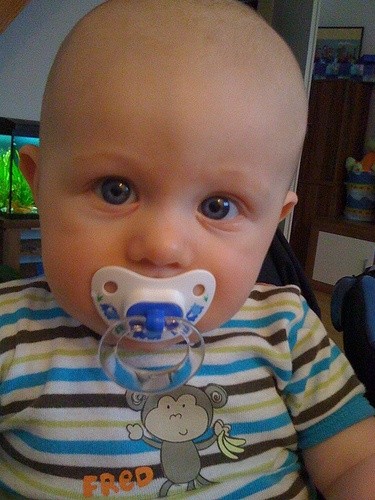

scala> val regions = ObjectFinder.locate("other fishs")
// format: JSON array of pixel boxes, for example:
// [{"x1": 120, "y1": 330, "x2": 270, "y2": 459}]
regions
[{"x1": 5, "y1": 196, "x2": 37, "y2": 214}]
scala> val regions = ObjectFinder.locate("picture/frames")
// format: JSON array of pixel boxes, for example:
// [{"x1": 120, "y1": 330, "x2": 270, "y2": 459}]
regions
[{"x1": 315, "y1": 26, "x2": 365, "y2": 64}]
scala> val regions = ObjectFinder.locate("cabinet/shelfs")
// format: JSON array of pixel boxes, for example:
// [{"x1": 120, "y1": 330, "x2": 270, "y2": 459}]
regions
[
  {"x1": 289, "y1": 78, "x2": 375, "y2": 294},
  {"x1": 0, "y1": 217, "x2": 41, "y2": 276}
]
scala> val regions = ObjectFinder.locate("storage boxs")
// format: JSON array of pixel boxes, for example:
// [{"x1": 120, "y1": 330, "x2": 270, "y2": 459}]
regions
[{"x1": 0, "y1": 117, "x2": 40, "y2": 219}]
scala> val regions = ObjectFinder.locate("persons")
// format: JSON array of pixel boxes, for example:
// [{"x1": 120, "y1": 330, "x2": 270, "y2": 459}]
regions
[{"x1": 0, "y1": 0, "x2": 375, "y2": 500}]
